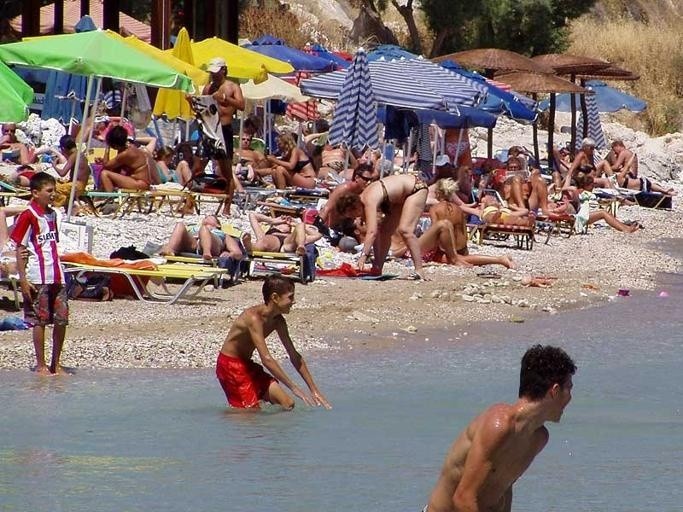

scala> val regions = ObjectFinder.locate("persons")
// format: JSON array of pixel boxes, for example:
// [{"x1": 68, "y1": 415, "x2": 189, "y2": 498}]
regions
[
  {"x1": 216, "y1": 275, "x2": 332, "y2": 413},
  {"x1": 10, "y1": 173, "x2": 71, "y2": 378},
  {"x1": 0, "y1": 59, "x2": 677, "y2": 281},
  {"x1": 422, "y1": 345, "x2": 577, "y2": 511}
]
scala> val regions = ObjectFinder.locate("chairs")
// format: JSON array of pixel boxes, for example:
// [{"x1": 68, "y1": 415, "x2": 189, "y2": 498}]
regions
[{"x1": 0, "y1": 134, "x2": 671, "y2": 310}]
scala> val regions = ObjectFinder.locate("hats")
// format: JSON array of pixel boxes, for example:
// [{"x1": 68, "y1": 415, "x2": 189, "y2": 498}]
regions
[
  {"x1": 435, "y1": 155, "x2": 451, "y2": 166},
  {"x1": 206, "y1": 58, "x2": 225, "y2": 74}
]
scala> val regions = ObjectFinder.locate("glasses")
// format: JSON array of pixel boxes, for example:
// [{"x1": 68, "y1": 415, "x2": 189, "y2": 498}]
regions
[{"x1": 359, "y1": 175, "x2": 373, "y2": 181}]
